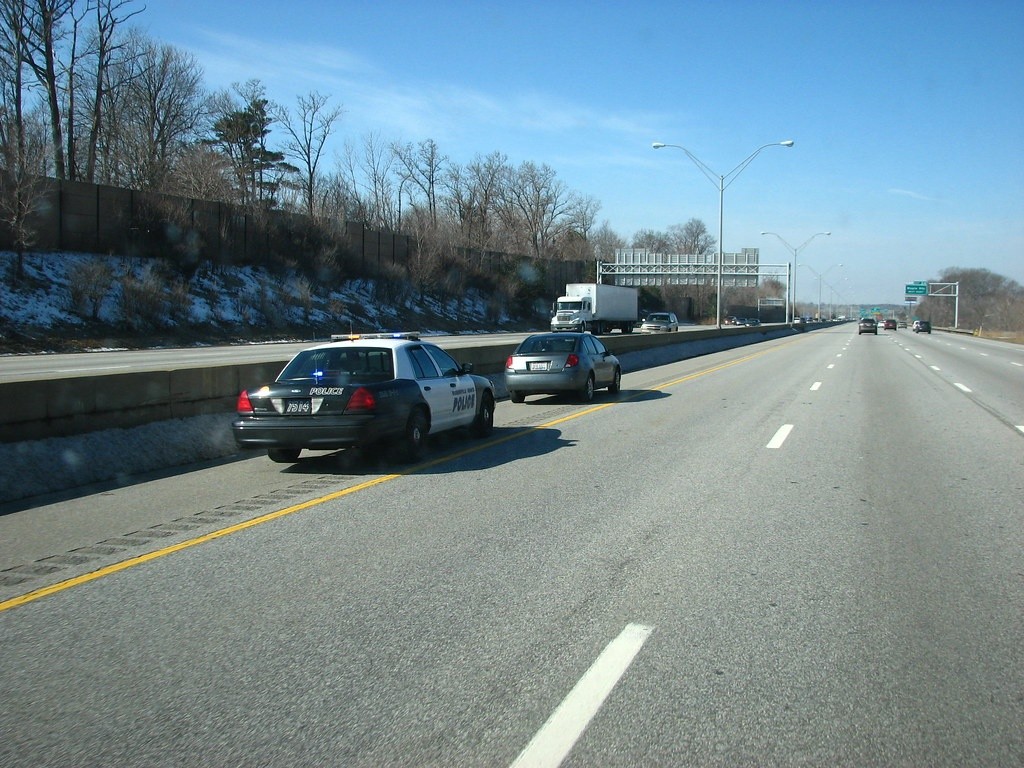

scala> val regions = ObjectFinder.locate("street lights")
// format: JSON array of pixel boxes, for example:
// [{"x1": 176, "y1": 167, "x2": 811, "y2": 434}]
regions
[
  {"x1": 760, "y1": 230, "x2": 831, "y2": 325},
  {"x1": 798, "y1": 263, "x2": 852, "y2": 323},
  {"x1": 651, "y1": 139, "x2": 796, "y2": 331}
]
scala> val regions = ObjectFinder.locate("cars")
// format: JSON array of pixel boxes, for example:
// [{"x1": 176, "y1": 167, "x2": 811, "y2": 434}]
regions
[
  {"x1": 736, "y1": 318, "x2": 748, "y2": 325},
  {"x1": 795, "y1": 317, "x2": 805, "y2": 324},
  {"x1": 915, "y1": 320, "x2": 931, "y2": 334},
  {"x1": 744, "y1": 317, "x2": 761, "y2": 327},
  {"x1": 724, "y1": 316, "x2": 737, "y2": 325},
  {"x1": 813, "y1": 318, "x2": 823, "y2": 322},
  {"x1": 884, "y1": 319, "x2": 896, "y2": 330},
  {"x1": 912, "y1": 320, "x2": 921, "y2": 331},
  {"x1": 504, "y1": 330, "x2": 623, "y2": 404},
  {"x1": 231, "y1": 331, "x2": 497, "y2": 465},
  {"x1": 897, "y1": 322, "x2": 907, "y2": 329},
  {"x1": 878, "y1": 320, "x2": 887, "y2": 328},
  {"x1": 806, "y1": 316, "x2": 813, "y2": 322}
]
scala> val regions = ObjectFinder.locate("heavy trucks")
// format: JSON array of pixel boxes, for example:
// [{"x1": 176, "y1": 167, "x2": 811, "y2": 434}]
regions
[{"x1": 549, "y1": 282, "x2": 641, "y2": 333}]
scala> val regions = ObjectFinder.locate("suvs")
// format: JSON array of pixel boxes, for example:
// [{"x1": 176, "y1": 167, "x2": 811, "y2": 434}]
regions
[
  {"x1": 640, "y1": 312, "x2": 680, "y2": 333},
  {"x1": 858, "y1": 318, "x2": 878, "y2": 335}
]
